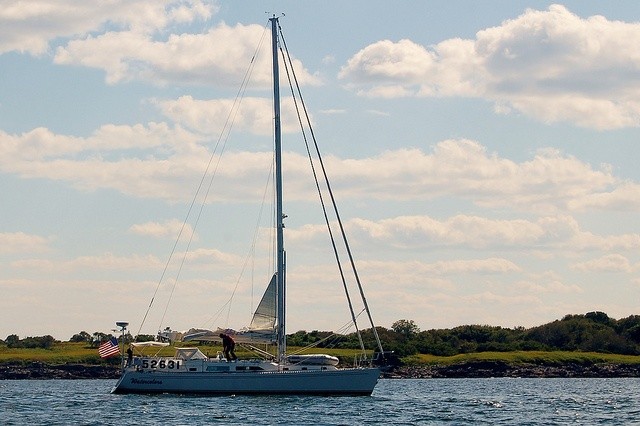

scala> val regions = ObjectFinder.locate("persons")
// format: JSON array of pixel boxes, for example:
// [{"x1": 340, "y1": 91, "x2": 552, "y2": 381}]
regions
[
  {"x1": 125, "y1": 344, "x2": 134, "y2": 368},
  {"x1": 219, "y1": 333, "x2": 237, "y2": 362}
]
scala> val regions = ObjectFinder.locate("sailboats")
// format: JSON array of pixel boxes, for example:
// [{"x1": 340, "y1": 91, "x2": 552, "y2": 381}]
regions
[{"x1": 110, "y1": 12, "x2": 381, "y2": 393}]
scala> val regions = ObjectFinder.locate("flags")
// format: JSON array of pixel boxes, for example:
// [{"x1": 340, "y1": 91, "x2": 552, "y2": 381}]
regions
[{"x1": 98, "y1": 338, "x2": 121, "y2": 359}]
